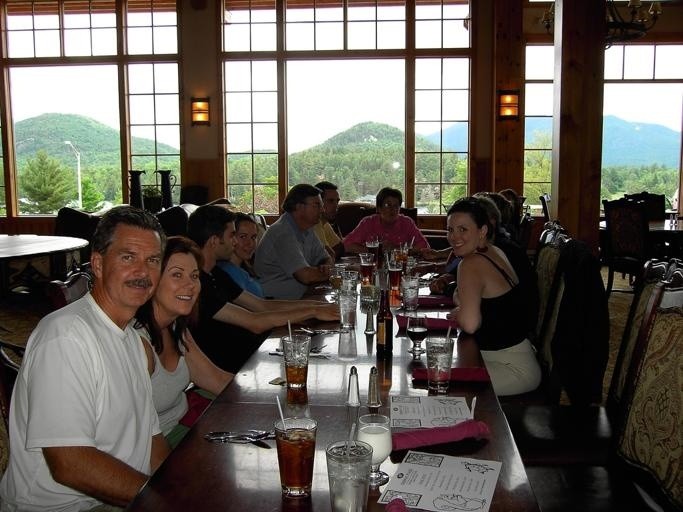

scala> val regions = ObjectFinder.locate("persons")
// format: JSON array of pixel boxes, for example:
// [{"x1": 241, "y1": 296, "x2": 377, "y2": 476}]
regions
[
  {"x1": 0, "y1": 206, "x2": 165, "y2": 510},
  {"x1": 418, "y1": 188, "x2": 542, "y2": 397},
  {"x1": 187, "y1": 180, "x2": 345, "y2": 373},
  {"x1": 139, "y1": 233, "x2": 235, "y2": 450},
  {"x1": 342, "y1": 186, "x2": 432, "y2": 256}
]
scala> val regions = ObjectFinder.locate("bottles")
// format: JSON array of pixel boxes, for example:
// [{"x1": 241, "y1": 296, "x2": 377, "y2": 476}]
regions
[
  {"x1": 364, "y1": 306, "x2": 377, "y2": 334},
  {"x1": 376, "y1": 287, "x2": 393, "y2": 353},
  {"x1": 669, "y1": 214, "x2": 678, "y2": 226},
  {"x1": 367, "y1": 366, "x2": 382, "y2": 409},
  {"x1": 376, "y1": 354, "x2": 396, "y2": 405},
  {"x1": 365, "y1": 336, "x2": 375, "y2": 360},
  {"x1": 346, "y1": 366, "x2": 360, "y2": 407}
]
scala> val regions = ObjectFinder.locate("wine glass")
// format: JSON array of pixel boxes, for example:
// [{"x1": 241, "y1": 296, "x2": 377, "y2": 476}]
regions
[
  {"x1": 406, "y1": 354, "x2": 428, "y2": 377},
  {"x1": 358, "y1": 414, "x2": 391, "y2": 486},
  {"x1": 407, "y1": 312, "x2": 430, "y2": 354}
]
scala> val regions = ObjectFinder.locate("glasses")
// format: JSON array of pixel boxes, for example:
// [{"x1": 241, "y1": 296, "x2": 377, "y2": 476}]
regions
[{"x1": 380, "y1": 203, "x2": 401, "y2": 211}]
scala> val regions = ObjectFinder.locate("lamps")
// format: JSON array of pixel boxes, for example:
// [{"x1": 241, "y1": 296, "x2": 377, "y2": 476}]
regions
[
  {"x1": 496, "y1": 88, "x2": 520, "y2": 122},
  {"x1": 188, "y1": 94, "x2": 210, "y2": 125}
]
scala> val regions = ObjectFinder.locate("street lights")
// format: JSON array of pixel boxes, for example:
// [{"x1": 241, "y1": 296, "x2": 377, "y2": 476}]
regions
[{"x1": 63, "y1": 140, "x2": 82, "y2": 209}]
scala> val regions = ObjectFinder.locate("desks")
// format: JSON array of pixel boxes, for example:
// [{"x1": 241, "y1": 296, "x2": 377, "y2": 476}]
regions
[{"x1": 127, "y1": 256, "x2": 539, "y2": 512}]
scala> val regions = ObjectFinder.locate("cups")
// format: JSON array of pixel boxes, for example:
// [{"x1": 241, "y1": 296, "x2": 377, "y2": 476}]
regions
[
  {"x1": 387, "y1": 246, "x2": 420, "y2": 311},
  {"x1": 281, "y1": 335, "x2": 311, "y2": 385},
  {"x1": 276, "y1": 417, "x2": 316, "y2": 498},
  {"x1": 326, "y1": 439, "x2": 374, "y2": 512},
  {"x1": 284, "y1": 386, "x2": 311, "y2": 418},
  {"x1": 328, "y1": 262, "x2": 358, "y2": 329},
  {"x1": 425, "y1": 335, "x2": 455, "y2": 397},
  {"x1": 279, "y1": 497, "x2": 315, "y2": 512},
  {"x1": 359, "y1": 238, "x2": 384, "y2": 305},
  {"x1": 337, "y1": 327, "x2": 358, "y2": 363}
]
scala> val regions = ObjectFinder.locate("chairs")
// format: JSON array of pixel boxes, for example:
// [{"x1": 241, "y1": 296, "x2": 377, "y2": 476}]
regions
[
  {"x1": 477, "y1": 190, "x2": 683, "y2": 512},
  {"x1": 0, "y1": 198, "x2": 246, "y2": 510}
]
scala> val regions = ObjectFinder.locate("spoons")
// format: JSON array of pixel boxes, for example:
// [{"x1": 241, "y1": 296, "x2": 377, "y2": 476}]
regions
[{"x1": 206, "y1": 429, "x2": 275, "y2": 443}]
